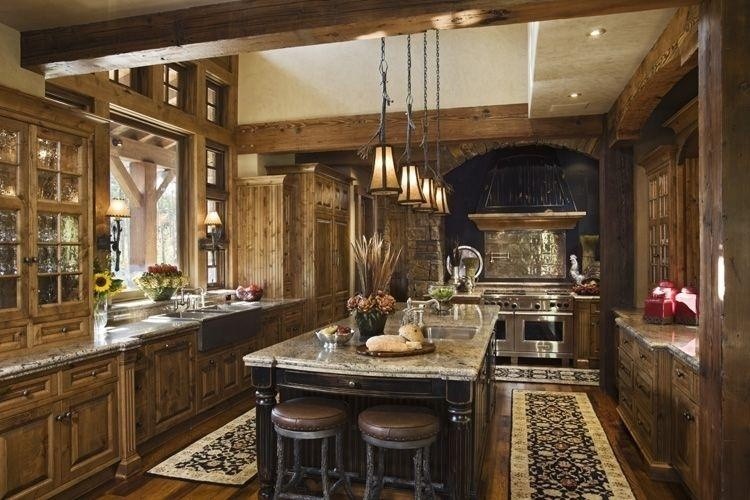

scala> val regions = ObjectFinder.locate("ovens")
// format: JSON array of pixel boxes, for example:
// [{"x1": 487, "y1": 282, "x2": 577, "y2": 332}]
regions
[{"x1": 493, "y1": 310, "x2": 574, "y2": 367}]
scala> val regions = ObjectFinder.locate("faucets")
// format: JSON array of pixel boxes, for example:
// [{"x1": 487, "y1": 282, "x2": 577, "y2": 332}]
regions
[{"x1": 181, "y1": 286, "x2": 204, "y2": 306}]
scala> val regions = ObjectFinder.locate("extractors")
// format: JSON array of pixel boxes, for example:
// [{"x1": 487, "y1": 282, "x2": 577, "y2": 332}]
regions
[{"x1": 467, "y1": 145, "x2": 588, "y2": 231}]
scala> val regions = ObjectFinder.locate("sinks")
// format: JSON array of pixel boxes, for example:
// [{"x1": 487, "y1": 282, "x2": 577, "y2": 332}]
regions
[{"x1": 163, "y1": 311, "x2": 225, "y2": 318}]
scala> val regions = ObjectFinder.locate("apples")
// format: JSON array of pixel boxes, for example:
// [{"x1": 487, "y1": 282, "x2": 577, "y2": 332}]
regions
[
  {"x1": 430, "y1": 288, "x2": 452, "y2": 299},
  {"x1": 236, "y1": 285, "x2": 262, "y2": 292}
]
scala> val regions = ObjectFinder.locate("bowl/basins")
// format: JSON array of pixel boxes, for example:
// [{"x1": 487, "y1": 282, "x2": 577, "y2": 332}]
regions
[
  {"x1": 235, "y1": 289, "x2": 264, "y2": 302},
  {"x1": 316, "y1": 349, "x2": 350, "y2": 365},
  {"x1": 453, "y1": 276, "x2": 476, "y2": 292},
  {"x1": 314, "y1": 329, "x2": 356, "y2": 349}
]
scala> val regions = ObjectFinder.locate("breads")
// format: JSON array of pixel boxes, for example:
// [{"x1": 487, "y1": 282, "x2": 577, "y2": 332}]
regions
[{"x1": 366, "y1": 324, "x2": 424, "y2": 352}]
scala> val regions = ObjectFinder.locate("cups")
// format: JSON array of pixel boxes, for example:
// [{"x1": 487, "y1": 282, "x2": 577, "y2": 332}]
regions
[
  {"x1": 0, "y1": 207, "x2": 20, "y2": 242},
  {"x1": 39, "y1": 245, "x2": 81, "y2": 274},
  {"x1": 37, "y1": 171, "x2": 79, "y2": 204},
  {"x1": 37, "y1": 138, "x2": 79, "y2": 173},
  {"x1": 0, "y1": 130, "x2": 18, "y2": 164},
  {"x1": 0, "y1": 246, "x2": 17, "y2": 275},
  {"x1": 37, "y1": 211, "x2": 78, "y2": 243},
  {"x1": 0, "y1": 167, "x2": 18, "y2": 196}
]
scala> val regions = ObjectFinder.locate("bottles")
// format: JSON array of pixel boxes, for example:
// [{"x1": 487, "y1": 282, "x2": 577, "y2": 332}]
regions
[{"x1": 226, "y1": 294, "x2": 231, "y2": 305}]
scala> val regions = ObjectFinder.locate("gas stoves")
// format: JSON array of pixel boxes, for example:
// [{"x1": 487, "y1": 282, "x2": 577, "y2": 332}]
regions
[{"x1": 483, "y1": 289, "x2": 574, "y2": 311}]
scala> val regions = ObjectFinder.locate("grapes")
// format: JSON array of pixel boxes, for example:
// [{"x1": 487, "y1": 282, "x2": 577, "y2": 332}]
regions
[{"x1": 337, "y1": 324, "x2": 350, "y2": 334}]
